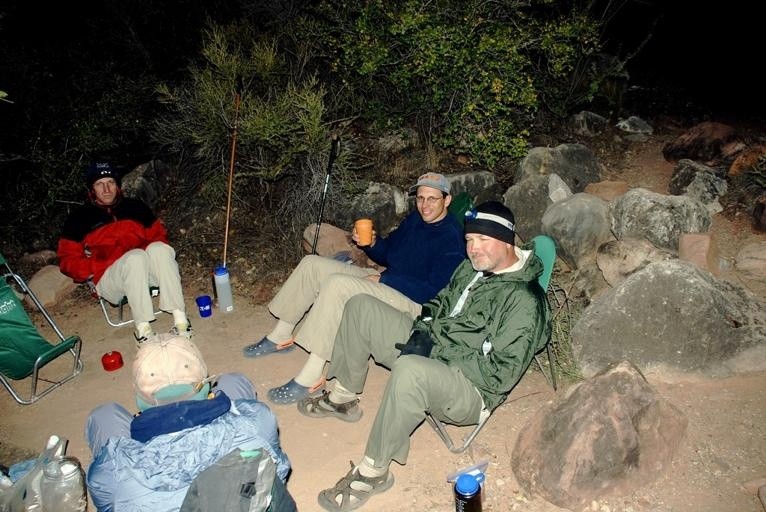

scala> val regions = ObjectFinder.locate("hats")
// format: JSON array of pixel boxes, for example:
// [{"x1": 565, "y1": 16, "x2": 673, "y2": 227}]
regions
[
  {"x1": 128, "y1": 332, "x2": 214, "y2": 406},
  {"x1": 86, "y1": 160, "x2": 117, "y2": 191},
  {"x1": 461, "y1": 198, "x2": 518, "y2": 248},
  {"x1": 408, "y1": 170, "x2": 453, "y2": 196}
]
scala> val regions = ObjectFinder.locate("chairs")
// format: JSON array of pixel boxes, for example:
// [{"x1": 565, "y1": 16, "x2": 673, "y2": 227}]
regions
[
  {"x1": 0, "y1": 253, "x2": 82, "y2": 406},
  {"x1": 422, "y1": 235, "x2": 559, "y2": 454},
  {"x1": 53, "y1": 197, "x2": 165, "y2": 327}
]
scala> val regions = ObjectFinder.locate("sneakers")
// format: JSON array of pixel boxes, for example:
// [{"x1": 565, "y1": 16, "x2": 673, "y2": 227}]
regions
[
  {"x1": 132, "y1": 328, "x2": 156, "y2": 349},
  {"x1": 172, "y1": 318, "x2": 197, "y2": 341}
]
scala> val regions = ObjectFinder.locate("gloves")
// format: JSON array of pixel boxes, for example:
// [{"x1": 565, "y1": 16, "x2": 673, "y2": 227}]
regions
[{"x1": 392, "y1": 329, "x2": 436, "y2": 362}]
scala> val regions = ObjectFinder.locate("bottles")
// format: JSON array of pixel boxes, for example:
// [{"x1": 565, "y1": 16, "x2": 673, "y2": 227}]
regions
[
  {"x1": 451, "y1": 471, "x2": 487, "y2": 512},
  {"x1": 39, "y1": 455, "x2": 87, "y2": 512},
  {"x1": 213, "y1": 268, "x2": 234, "y2": 314}
]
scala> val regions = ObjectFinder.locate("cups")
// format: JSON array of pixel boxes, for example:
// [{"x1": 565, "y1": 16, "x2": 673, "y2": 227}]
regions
[
  {"x1": 355, "y1": 219, "x2": 372, "y2": 247},
  {"x1": 196, "y1": 295, "x2": 212, "y2": 318}
]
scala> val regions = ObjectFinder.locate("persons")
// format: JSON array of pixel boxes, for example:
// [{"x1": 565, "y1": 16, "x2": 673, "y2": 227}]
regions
[
  {"x1": 296, "y1": 202, "x2": 552, "y2": 512},
  {"x1": 58, "y1": 161, "x2": 194, "y2": 348},
  {"x1": 243, "y1": 173, "x2": 469, "y2": 405},
  {"x1": 85, "y1": 335, "x2": 293, "y2": 511}
]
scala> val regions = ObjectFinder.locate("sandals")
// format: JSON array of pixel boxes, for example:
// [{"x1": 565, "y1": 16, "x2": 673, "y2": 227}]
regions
[
  {"x1": 296, "y1": 390, "x2": 364, "y2": 423},
  {"x1": 266, "y1": 375, "x2": 328, "y2": 407},
  {"x1": 240, "y1": 333, "x2": 297, "y2": 358},
  {"x1": 317, "y1": 463, "x2": 395, "y2": 512}
]
